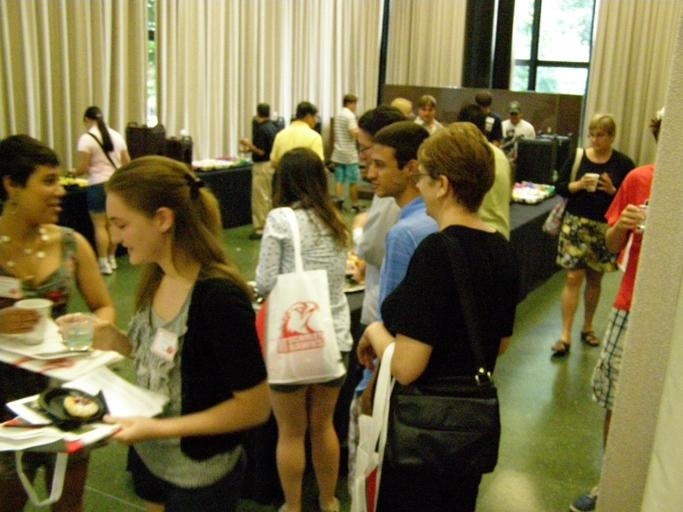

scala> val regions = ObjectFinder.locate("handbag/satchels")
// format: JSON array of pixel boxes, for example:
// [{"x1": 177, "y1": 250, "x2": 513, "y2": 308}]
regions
[
  {"x1": 348, "y1": 414, "x2": 386, "y2": 512},
  {"x1": 265, "y1": 269, "x2": 348, "y2": 387},
  {"x1": 387, "y1": 368, "x2": 500, "y2": 474},
  {"x1": 542, "y1": 198, "x2": 569, "y2": 236},
  {"x1": 0, "y1": 346, "x2": 171, "y2": 509}
]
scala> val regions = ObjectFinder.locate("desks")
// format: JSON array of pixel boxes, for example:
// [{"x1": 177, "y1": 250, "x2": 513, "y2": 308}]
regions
[
  {"x1": 57, "y1": 154, "x2": 254, "y2": 262},
  {"x1": 204, "y1": 183, "x2": 569, "y2": 510}
]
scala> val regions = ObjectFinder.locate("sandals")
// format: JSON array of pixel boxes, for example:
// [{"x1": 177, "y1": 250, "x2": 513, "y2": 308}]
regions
[
  {"x1": 578, "y1": 331, "x2": 600, "y2": 347},
  {"x1": 551, "y1": 337, "x2": 571, "y2": 353}
]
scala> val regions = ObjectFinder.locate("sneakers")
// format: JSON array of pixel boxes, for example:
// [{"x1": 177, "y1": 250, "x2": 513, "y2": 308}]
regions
[
  {"x1": 569, "y1": 483, "x2": 601, "y2": 512},
  {"x1": 98, "y1": 257, "x2": 118, "y2": 274}
]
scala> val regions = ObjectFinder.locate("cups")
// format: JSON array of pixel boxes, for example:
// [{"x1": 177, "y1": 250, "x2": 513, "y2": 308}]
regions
[
  {"x1": 16, "y1": 299, "x2": 51, "y2": 344},
  {"x1": 584, "y1": 174, "x2": 600, "y2": 192},
  {"x1": 60, "y1": 317, "x2": 97, "y2": 351}
]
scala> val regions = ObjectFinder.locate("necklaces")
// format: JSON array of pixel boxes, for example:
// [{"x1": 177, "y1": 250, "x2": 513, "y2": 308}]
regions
[
  {"x1": 0, "y1": 225, "x2": 45, "y2": 255},
  {"x1": 1, "y1": 228, "x2": 49, "y2": 281}
]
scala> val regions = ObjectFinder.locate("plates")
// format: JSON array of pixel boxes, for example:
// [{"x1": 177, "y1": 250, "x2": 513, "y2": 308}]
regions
[{"x1": 38, "y1": 387, "x2": 104, "y2": 426}]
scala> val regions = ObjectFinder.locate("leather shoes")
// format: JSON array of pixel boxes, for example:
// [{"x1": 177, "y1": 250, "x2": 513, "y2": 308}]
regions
[{"x1": 249, "y1": 232, "x2": 262, "y2": 240}]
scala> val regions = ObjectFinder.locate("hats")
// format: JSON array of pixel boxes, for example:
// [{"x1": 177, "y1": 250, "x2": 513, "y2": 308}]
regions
[{"x1": 507, "y1": 99, "x2": 523, "y2": 115}]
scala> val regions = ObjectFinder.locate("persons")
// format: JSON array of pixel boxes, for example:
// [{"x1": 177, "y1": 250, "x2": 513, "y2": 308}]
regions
[
  {"x1": 55, "y1": 155, "x2": 274, "y2": 511},
  {"x1": 252, "y1": 148, "x2": 353, "y2": 511},
  {"x1": 66, "y1": 107, "x2": 132, "y2": 273},
  {"x1": 543, "y1": 116, "x2": 634, "y2": 357},
  {"x1": 1, "y1": 135, "x2": 114, "y2": 512},
  {"x1": 237, "y1": 91, "x2": 577, "y2": 240},
  {"x1": 570, "y1": 110, "x2": 667, "y2": 512},
  {"x1": 346, "y1": 107, "x2": 403, "y2": 341},
  {"x1": 356, "y1": 121, "x2": 525, "y2": 512},
  {"x1": 356, "y1": 121, "x2": 430, "y2": 395}
]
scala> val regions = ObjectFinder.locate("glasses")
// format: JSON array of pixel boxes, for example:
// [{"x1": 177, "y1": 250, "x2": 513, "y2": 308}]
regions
[
  {"x1": 587, "y1": 133, "x2": 605, "y2": 138},
  {"x1": 354, "y1": 140, "x2": 373, "y2": 153},
  {"x1": 411, "y1": 168, "x2": 432, "y2": 182}
]
